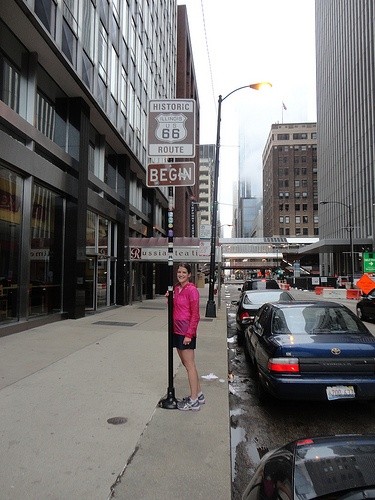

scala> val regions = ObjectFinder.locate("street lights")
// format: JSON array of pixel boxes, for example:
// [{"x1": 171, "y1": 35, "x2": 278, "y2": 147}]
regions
[
  {"x1": 218, "y1": 224, "x2": 232, "y2": 238},
  {"x1": 319, "y1": 201, "x2": 355, "y2": 289},
  {"x1": 206, "y1": 81, "x2": 273, "y2": 317}
]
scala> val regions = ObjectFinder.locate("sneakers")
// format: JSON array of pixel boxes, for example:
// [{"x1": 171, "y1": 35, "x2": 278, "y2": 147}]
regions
[
  {"x1": 176, "y1": 399, "x2": 199, "y2": 411},
  {"x1": 182, "y1": 393, "x2": 205, "y2": 404}
]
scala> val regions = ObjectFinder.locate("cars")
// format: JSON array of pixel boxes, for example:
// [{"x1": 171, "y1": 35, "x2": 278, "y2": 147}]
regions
[
  {"x1": 242, "y1": 299, "x2": 375, "y2": 402},
  {"x1": 243, "y1": 434, "x2": 375, "y2": 500},
  {"x1": 231, "y1": 279, "x2": 297, "y2": 337},
  {"x1": 356, "y1": 288, "x2": 375, "y2": 324}
]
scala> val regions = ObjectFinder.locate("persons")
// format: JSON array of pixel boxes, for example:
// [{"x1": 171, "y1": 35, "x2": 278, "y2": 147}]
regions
[
  {"x1": 335, "y1": 270, "x2": 347, "y2": 289},
  {"x1": 166, "y1": 262, "x2": 206, "y2": 412}
]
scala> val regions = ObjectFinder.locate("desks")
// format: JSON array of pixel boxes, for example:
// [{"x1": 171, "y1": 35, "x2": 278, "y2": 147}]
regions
[{"x1": 3, "y1": 285, "x2": 61, "y2": 317}]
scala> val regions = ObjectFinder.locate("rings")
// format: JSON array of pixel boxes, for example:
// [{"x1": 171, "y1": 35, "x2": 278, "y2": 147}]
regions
[{"x1": 188, "y1": 343, "x2": 189, "y2": 344}]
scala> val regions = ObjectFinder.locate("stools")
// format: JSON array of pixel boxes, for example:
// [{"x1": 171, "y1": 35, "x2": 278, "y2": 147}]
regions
[{"x1": 0, "y1": 285, "x2": 8, "y2": 320}]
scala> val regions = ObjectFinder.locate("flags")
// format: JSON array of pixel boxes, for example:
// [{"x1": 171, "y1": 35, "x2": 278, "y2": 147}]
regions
[{"x1": 283, "y1": 103, "x2": 287, "y2": 110}]
